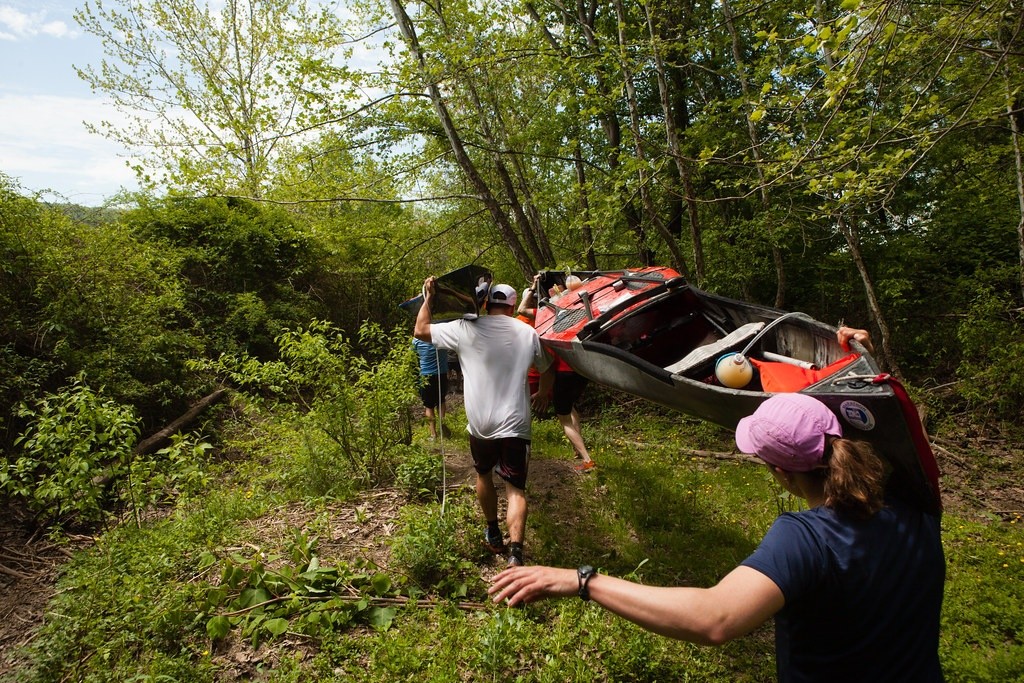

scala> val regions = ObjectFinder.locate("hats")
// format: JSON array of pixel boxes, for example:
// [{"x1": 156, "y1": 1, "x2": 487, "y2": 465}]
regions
[
  {"x1": 735, "y1": 392, "x2": 844, "y2": 474},
  {"x1": 488, "y1": 284, "x2": 518, "y2": 306}
]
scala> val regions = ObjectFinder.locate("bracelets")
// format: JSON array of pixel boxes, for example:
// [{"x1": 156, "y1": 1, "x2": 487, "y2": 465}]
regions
[{"x1": 528, "y1": 289, "x2": 535, "y2": 292}]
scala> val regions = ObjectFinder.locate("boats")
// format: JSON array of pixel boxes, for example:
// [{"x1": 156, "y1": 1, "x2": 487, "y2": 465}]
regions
[
  {"x1": 400, "y1": 265, "x2": 494, "y2": 317},
  {"x1": 535, "y1": 266, "x2": 943, "y2": 483}
]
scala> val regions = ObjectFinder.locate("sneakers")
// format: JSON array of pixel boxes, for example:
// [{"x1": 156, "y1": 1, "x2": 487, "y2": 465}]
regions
[
  {"x1": 483, "y1": 528, "x2": 503, "y2": 552},
  {"x1": 574, "y1": 453, "x2": 580, "y2": 458},
  {"x1": 506, "y1": 556, "x2": 524, "y2": 569},
  {"x1": 574, "y1": 460, "x2": 597, "y2": 473}
]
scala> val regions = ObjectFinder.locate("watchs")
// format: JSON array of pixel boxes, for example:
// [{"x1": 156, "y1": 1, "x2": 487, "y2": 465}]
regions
[{"x1": 576, "y1": 564, "x2": 598, "y2": 601}]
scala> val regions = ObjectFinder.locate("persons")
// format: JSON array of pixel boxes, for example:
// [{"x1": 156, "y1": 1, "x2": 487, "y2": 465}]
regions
[
  {"x1": 517, "y1": 287, "x2": 550, "y2": 423},
  {"x1": 412, "y1": 336, "x2": 452, "y2": 442},
  {"x1": 414, "y1": 275, "x2": 557, "y2": 568},
  {"x1": 517, "y1": 269, "x2": 596, "y2": 473},
  {"x1": 487, "y1": 325, "x2": 948, "y2": 683}
]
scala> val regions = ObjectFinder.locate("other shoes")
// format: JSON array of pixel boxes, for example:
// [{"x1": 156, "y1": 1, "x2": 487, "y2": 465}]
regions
[
  {"x1": 429, "y1": 435, "x2": 436, "y2": 439},
  {"x1": 441, "y1": 425, "x2": 451, "y2": 439}
]
